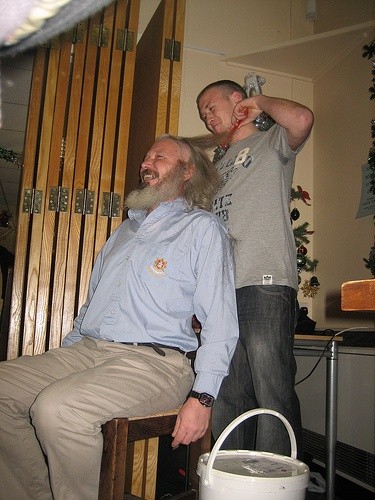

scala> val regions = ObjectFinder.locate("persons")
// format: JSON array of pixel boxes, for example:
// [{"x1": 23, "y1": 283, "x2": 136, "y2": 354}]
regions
[
  {"x1": 0, "y1": 137, "x2": 239, "y2": 500},
  {"x1": 196, "y1": 80, "x2": 315, "y2": 460}
]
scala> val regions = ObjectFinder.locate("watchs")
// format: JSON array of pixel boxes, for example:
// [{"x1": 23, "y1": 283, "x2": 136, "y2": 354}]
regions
[{"x1": 190, "y1": 391, "x2": 215, "y2": 407}]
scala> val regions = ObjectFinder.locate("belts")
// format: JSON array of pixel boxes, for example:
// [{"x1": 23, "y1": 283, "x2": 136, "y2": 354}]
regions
[{"x1": 120, "y1": 341, "x2": 193, "y2": 360}]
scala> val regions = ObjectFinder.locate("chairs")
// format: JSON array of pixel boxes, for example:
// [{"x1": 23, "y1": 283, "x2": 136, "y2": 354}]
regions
[{"x1": 100, "y1": 398, "x2": 214, "y2": 500}]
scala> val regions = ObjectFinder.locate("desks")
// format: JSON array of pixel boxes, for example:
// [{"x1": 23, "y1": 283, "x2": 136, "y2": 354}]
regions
[{"x1": 289, "y1": 335, "x2": 343, "y2": 500}]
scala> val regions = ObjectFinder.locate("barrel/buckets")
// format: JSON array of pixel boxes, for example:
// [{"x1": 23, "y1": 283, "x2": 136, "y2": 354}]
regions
[{"x1": 196, "y1": 408, "x2": 308, "y2": 500}]
